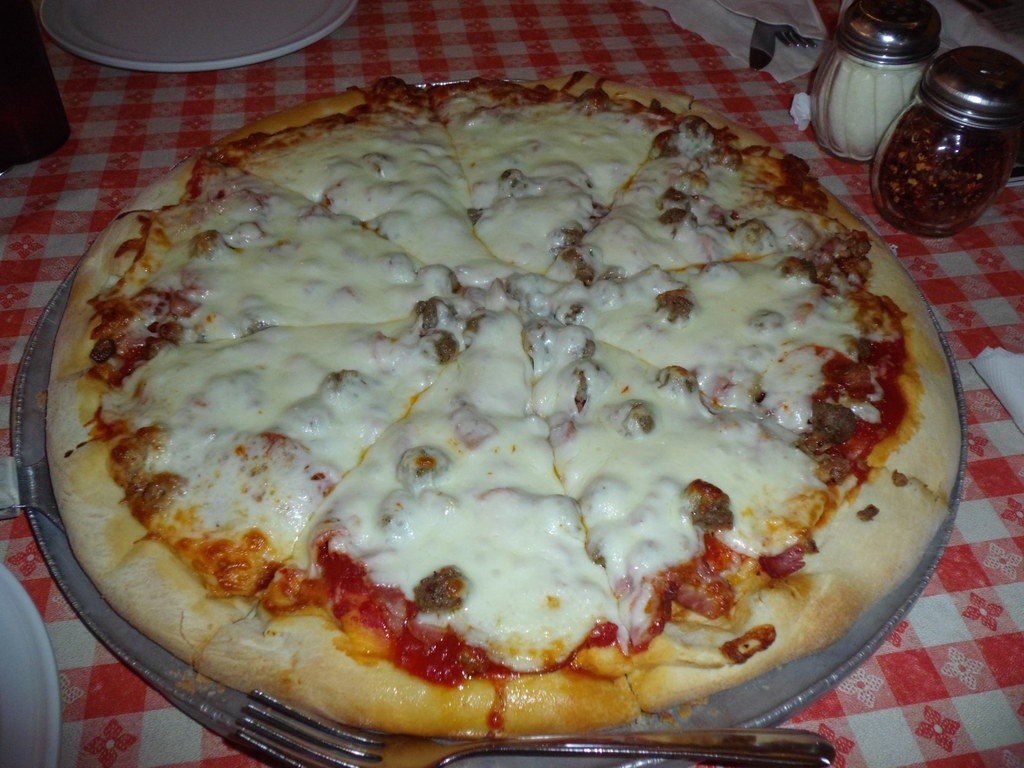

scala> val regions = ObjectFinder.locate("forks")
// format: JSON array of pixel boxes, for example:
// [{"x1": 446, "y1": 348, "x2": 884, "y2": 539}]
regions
[
  {"x1": 776, "y1": 25, "x2": 817, "y2": 49},
  {"x1": 235, "y1": 689, "x2": 836, "y2": 768}
]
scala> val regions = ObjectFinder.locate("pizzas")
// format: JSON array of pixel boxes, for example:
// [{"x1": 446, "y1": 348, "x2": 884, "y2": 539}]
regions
[{"x1": 43, "y1": 65, "x2": 962, "y2": 742}]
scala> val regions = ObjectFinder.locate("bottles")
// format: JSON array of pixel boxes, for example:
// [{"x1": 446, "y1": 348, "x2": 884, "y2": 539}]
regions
[
  {"x1": 870, "y1": 45, "x2": 1024, "y2": 236},
  {"x1": 810, "y1": 0, "x2": 942, "y2": 164}
]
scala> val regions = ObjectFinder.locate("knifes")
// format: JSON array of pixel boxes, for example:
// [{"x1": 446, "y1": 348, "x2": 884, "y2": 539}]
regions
[{"x1": 750, "y1": 21, "x2": 776, "y2": 70}]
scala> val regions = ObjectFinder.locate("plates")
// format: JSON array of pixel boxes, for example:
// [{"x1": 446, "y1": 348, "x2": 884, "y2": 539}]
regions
[
  {"x1": 38, "y1": 0, "x2": 358, "y2": 72},
  {"x1": 0, "y1": 565, "x2": 62, "y2": 768},
  {"x1": 7, "y1": 79, "x2": 968, "y2": 768}
]
